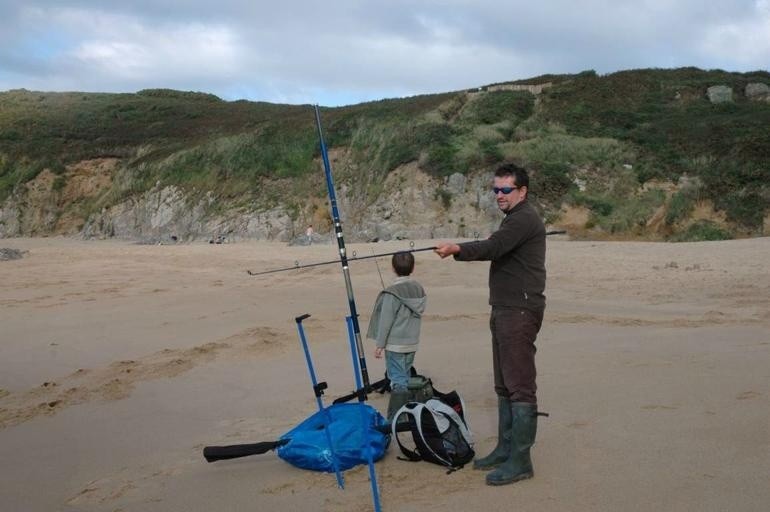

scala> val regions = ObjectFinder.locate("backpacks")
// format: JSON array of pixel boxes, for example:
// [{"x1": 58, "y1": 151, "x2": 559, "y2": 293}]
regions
[{"x1": 392, "y1": 396, "x2": 474, "y2": 471}]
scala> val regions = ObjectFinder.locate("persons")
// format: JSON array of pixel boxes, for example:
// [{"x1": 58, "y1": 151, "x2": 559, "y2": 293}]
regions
[
  {"x1": 434, "y1": 163, "x2": 546, "y2": 484},
  {"x1": 306, "y1": 224, "x2": 315, "y2": 246},
  {"x1": 365, "y1": 252, "x2": 427, "y2": 394}
]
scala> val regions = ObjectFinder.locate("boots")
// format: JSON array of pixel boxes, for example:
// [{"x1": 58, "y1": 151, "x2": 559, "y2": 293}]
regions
[{"x1": 474, "y1": 397, "x2": 537, "y2": 485}]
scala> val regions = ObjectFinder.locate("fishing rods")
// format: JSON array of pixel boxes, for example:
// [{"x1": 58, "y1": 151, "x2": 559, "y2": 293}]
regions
[
  {"x1": 313, "y1": 104, "x2": 372, "y2": 392},
  {"x1": 246, "y1": 231, "x2": 566, "y2": 275},
  {"x1": 373, "y1": 247, "x2": 386, "y2": 290}
]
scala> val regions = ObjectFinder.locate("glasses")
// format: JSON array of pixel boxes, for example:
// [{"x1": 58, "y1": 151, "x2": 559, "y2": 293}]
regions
[{"x1": 493, "y1": 187, "x2": 517, "y2": 194}]
